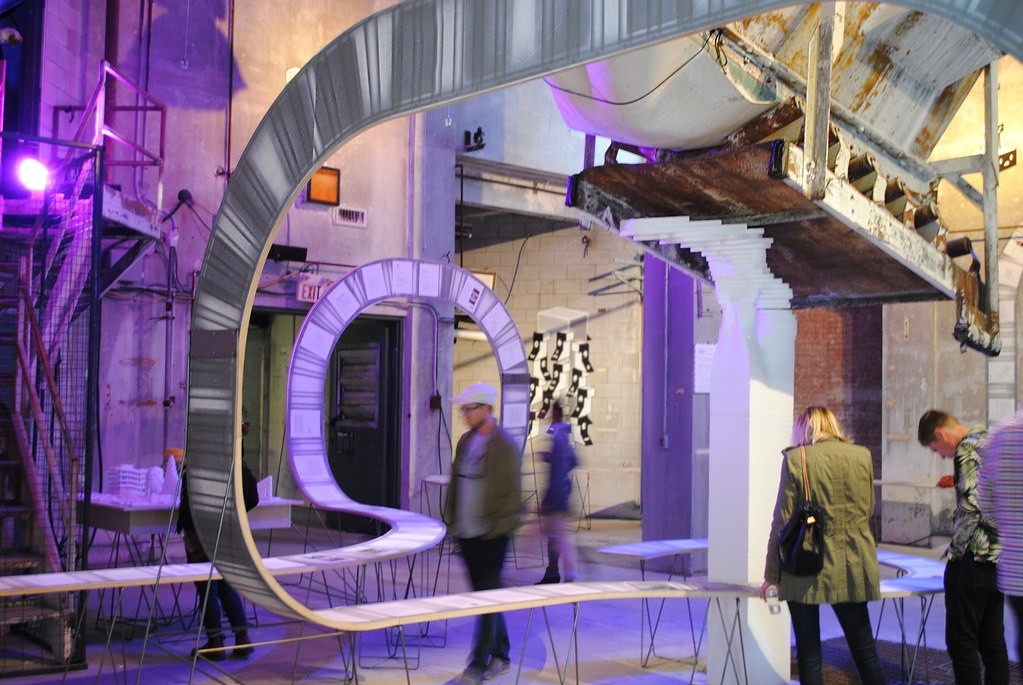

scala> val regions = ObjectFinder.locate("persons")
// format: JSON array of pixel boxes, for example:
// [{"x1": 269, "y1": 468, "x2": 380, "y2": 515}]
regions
[
  {"x1": 444, "y1": 383, "x2": 522, "y2": 685},
  {"x1": 535, "y1": 423, "x2": 578, "y2": 586},
  {"x1": 917, "y1": 411, "x2": 1010, "y2": 685},
  {"x1": 759, "y1": 405, "x2": 886, "y2": 685},
  {"x1": 977, "y1": 408, "x2": 1023, "y2": 679},
  {"x1": 173, "y1": 452, "x2": 258, "y2": 662}
]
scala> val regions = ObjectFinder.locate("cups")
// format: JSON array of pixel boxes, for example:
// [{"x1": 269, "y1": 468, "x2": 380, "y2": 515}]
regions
[{"x1": 765, "y1": 588, "x2": 781, "y2": 615}]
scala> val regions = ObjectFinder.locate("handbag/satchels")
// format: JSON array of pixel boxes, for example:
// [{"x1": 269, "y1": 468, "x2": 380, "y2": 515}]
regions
[{"x1": 773, "y1": 445, "x2": 826, "y2": 577}]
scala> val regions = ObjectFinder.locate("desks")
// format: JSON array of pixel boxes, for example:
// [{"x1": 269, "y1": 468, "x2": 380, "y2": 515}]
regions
[{"x1": 76, "y1": 494, "x2": 302, "y2": 641}]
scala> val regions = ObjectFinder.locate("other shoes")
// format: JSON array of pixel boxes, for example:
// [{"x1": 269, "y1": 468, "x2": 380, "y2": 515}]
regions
[
  {"x1": 191, "y1": 639, "x2": 224, "y2": 661},
  {"x1": 232, "y1": 632, "x2": 255, "y2": 656},
  {"x1": 534, "y1": 566, "x2": 561, "y2": 585},
  {"x1": 442, "y1": 668, "x2": 483, "y2": 685},
  {"x1": 482, "y1": 658, "x2": 510, "y2": 679}
]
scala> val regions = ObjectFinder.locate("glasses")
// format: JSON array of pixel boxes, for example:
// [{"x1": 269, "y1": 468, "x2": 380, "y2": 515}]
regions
[{"x1": 461, "y1": 403, "x2": 485, "y2": 415}]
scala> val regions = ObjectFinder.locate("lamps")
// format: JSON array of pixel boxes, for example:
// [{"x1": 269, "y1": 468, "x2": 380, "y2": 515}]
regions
[{"x1": 303, "y1": 166, "x2": 341, "y2": 205}]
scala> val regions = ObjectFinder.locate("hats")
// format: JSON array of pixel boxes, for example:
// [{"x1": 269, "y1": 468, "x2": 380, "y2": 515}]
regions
[{"x1": 447, "y1": 383, "x2": 496, "y2": 407}]
